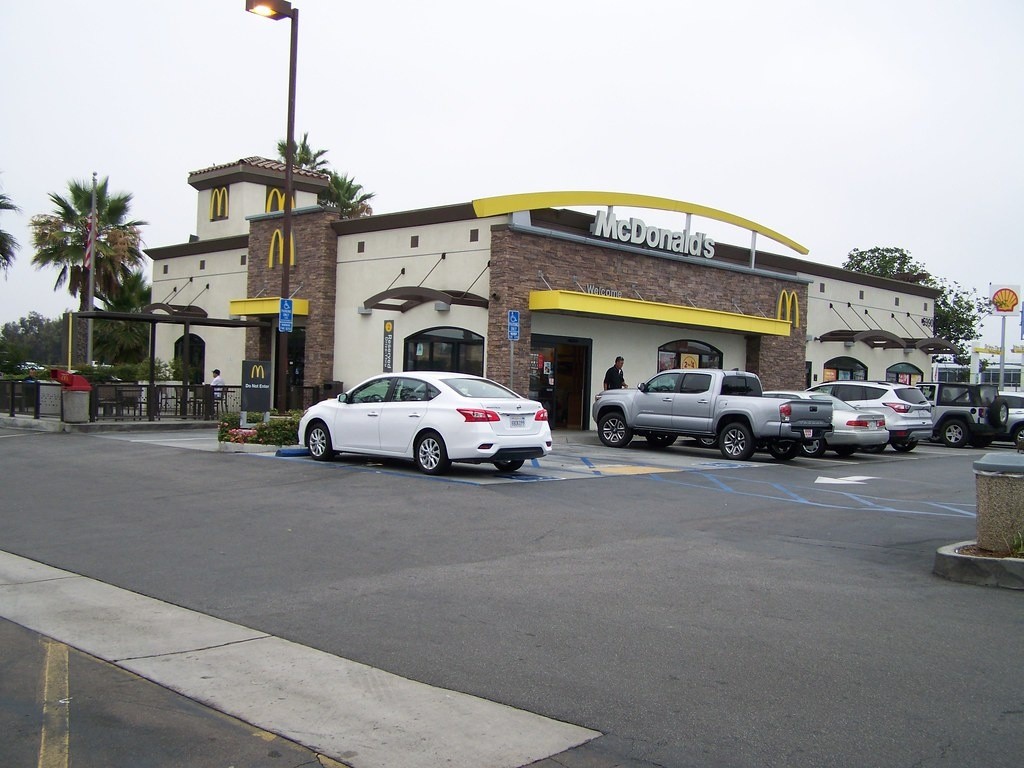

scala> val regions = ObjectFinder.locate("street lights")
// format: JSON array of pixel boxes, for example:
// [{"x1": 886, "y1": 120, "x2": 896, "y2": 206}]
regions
[{"x1": 246, "y1": 0, "x2": 298, "y2": 417}]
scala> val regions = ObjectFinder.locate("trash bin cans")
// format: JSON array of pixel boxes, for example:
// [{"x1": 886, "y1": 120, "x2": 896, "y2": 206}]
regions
[
  {"x1": 972, "y1": 451, "x2": 1024, "y2": 554},
  {"x1": 50, "y1": 368, "x2": 93, "y2": 423},
  {"x1": 323, "y1": 380, "x2": 344, "y2": 398}
]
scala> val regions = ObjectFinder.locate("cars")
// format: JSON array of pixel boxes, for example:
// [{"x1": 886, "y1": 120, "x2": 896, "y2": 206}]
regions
[
  {"x1": 762, "y1": 389, "x2": 890, "y2": 459},
  {"x1": 298, "y1": 369, "x2": 553, "y2": 476},
  {"x1": 987, "y1": 390, "x2": 1024, "y2": 449}
]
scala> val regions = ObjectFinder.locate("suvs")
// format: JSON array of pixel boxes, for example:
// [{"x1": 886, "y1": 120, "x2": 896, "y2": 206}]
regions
[
  {"x1": 916, "y1": 379, "x2": 1009, "y2": 448},
  {"x1": 804, "y1": 379, "x2": 934, "y2": 455}
]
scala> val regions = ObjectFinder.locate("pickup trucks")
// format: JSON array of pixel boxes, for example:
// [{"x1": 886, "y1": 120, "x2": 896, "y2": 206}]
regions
[
  {"x1": 591, "y1": 368, "x2": 833, "y2": 461},
  {"x1": 86, "y1": 360, "x2": 115, "y2": 372}
]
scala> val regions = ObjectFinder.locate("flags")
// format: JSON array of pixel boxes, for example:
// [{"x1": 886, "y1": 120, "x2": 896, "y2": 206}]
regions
[{"x1": 84, "y1": 196, "x2": 99, "y2": 271}]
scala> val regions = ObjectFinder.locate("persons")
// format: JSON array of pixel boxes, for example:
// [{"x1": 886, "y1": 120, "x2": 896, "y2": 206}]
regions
[
  {"x1": 23, "y1": 368, "x2": 37, "y2": 402},
  {"x1": 557, "y1": 368, "x2": 571, "y2": 424},
  {"x1": 208, "y1": 370, "x2": 224, "y2": 418},
  {"x1": 604, "y1": 356, "x2": 628, "y2": 392}
]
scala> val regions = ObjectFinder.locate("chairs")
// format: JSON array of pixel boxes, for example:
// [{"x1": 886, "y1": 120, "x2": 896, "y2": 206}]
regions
[
  {"x1": 95, "y1": 380, "x2": 144, "y2": 421},
  {"x1": 5, "y1": 379, "x2": 29, "y2": 413},
  {"x1": 158, "y1": 385, "x2": 228, "y2": 421}
]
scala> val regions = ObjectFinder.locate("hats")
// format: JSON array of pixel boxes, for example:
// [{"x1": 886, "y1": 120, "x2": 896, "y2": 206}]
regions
[{"x1": 212, "y1": 369, "x2": 220, "y2": 375}]
